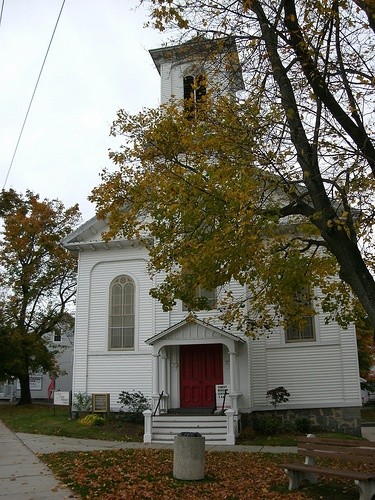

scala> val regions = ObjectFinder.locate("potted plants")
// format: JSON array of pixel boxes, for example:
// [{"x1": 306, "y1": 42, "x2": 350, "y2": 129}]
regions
[{"x1": 173, "y1": 432, "x2": 206, "y2": 480}]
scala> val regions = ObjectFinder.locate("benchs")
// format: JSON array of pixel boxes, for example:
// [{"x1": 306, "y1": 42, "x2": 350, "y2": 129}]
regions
[{"x1": 275, "y1": 433, "x2": 375, "y2": 500}]
